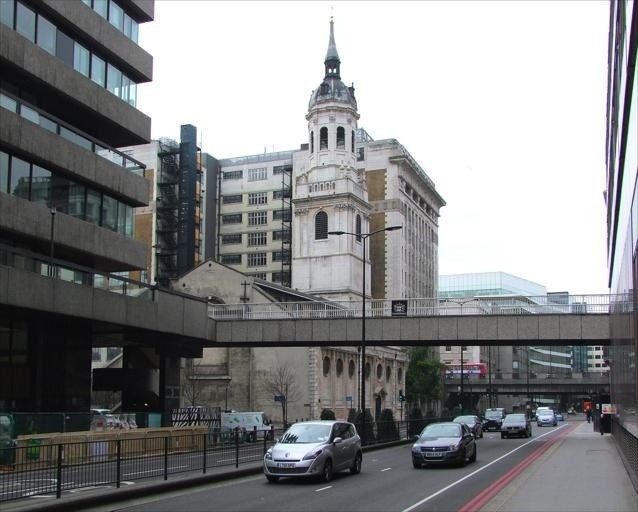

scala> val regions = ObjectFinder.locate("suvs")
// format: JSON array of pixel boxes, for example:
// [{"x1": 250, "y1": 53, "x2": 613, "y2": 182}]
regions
[{"x1": 482, "y1": 407, "x2": 506, "y2": 432}]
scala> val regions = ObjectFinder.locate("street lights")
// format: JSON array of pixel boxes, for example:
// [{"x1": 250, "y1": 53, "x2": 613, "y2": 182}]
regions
[
  {"x1": 440, "y1": 299, "x2": 478, "y2": 416},
  {"x1": 327, "y1": 226, "x2": 402, "y2": 446}
]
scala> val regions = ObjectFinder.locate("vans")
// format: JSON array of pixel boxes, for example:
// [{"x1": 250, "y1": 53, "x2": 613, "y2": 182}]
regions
[{"x1": 90, "y1": 409, "x2": 114, "y2": 427}]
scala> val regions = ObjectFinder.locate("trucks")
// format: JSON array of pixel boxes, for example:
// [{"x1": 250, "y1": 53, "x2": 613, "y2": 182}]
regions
[{"x1": 221, "y1": 412, "x2": 273, "y2": 443}]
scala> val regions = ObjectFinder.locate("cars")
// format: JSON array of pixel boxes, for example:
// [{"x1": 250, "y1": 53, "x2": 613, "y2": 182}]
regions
[
  {"x1": 264, "y1": 420, "x2": 363, "y2": 481},
  {"x1": 535, "y1": 407, "x2": 563, "y2": 427},
  {"x1": 453, "y1": 415, "x2": 483, "y2": 440},
  {"x1": 412, "y1": 422, "x2": 476, "y2": 468},
  {"x1": 501, "y1": 414, "x2": 531, "y2": 438}
]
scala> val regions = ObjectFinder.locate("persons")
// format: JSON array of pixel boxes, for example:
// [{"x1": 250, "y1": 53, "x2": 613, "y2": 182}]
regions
[{"x1": 585, "y1": 406, "x2": 592, "y2": 424}]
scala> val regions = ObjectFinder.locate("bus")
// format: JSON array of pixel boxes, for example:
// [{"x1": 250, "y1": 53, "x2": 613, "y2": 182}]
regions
[{"x1": 445, "y1": 363, "x2": 487, "y2": 380}]
